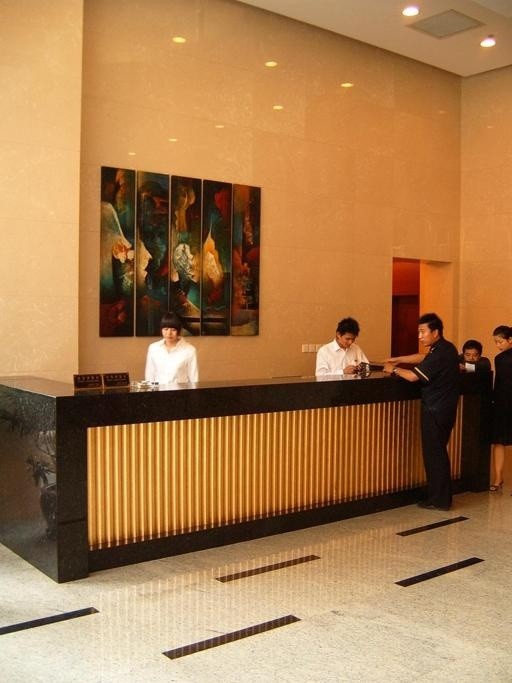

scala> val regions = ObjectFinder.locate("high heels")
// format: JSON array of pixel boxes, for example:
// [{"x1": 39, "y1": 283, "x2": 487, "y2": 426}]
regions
[{"x1": 490, "y1": 480, "x2": 504, "y2": 491}]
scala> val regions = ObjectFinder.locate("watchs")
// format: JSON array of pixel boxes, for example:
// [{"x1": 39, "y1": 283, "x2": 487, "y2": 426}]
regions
[{"x1": 392, "y1": 367, "x2": 400, "y2": 374}]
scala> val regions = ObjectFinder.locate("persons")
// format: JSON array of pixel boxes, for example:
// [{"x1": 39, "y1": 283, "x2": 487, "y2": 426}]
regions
[
  {"x1": 144, "y1": 310, "x2": 198, "y2": 384},
  {"x1": 380, "y1": 313, "x2": 461, "y2": 511},
  {"x1": 458, "y1": 341, "x2": 491, "y2": 373},
  {"x1": 314, "y1": 317, "x2": 369, "y2": 377},
  {"x1": 489, "y1": 325, "x2": 512, "y2": 492}
]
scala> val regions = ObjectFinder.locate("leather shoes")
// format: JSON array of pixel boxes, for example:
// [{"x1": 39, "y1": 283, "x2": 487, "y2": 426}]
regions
[
  {"x1": 471, "y1": 485, "x2": 489, "y2": 492},
  {"x1": 414, "y1": 490, "x2": 452, "y2": 511}
]
